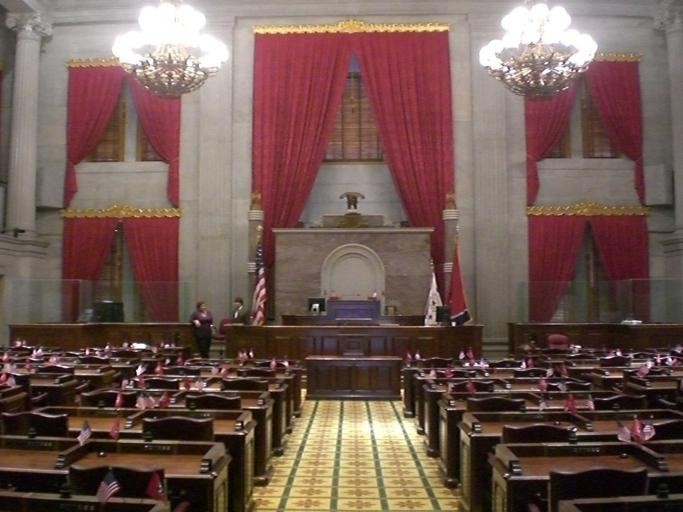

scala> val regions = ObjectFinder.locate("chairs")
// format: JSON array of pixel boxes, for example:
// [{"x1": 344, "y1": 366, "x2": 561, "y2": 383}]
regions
[
  {"x1": 1, "y1": 334, "x2": 308, "y2": 511},
  {"x1": 399, "y1": 348, "x2": 683, "y2": 511}
]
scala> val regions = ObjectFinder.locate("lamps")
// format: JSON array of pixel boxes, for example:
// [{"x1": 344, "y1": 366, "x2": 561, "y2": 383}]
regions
[
  {"x1": 109, "y1": 0, "x2": 233, "y2": 96},
  {"x1": 476, "y1": 0, "x2": 601, "y2": 100}
]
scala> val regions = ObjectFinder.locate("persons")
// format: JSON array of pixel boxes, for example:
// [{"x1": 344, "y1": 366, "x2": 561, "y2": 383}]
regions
[
  {"x1": 232, "y1": 296, "x2": 250, "y2": 326},
  {"x1": 190, "y1": 300, "x2": 214, "y2": 359}
]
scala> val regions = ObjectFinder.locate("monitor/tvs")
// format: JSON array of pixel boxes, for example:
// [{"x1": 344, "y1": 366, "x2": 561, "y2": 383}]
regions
[
  {"x1": 436, "y1": 305, "x2": 450, "y2": 322},
  {"x1": 308, "y1": 297, "x2": 325, "y2": 311}
]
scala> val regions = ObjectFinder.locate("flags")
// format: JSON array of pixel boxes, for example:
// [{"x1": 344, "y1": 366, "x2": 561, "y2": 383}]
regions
[
  {"x1": 445, "y1": 234, "x2": 472, "y2": 328},
  {"x1": 0, "y1": 333, "x2": 290, "y2": 505},
  {"x1": 424, "y1": 272, "x2": 445, "y2": 327},
  {"x1": 250, "y1": 237, "x2": 268, "y2": 326},
  {"x1": 404, "y1": 341, "x2": 682, "y2": 446}
]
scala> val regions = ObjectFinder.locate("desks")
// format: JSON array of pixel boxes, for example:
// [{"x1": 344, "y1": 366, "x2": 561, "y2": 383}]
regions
[{"x1": 305, "y1": 354, "x2": 402, "y2": 400}]
